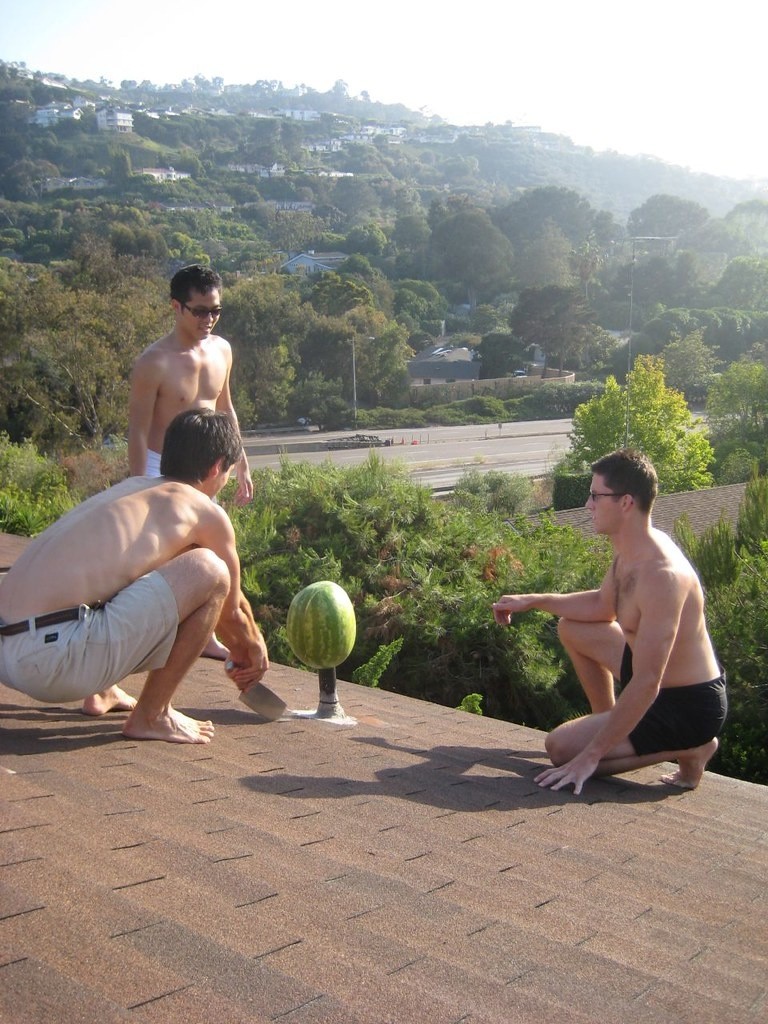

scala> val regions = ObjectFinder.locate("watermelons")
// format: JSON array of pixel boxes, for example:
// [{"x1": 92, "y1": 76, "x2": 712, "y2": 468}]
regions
[{"x1": 286, "y1": 581, "x2": 357, "y2": 669}]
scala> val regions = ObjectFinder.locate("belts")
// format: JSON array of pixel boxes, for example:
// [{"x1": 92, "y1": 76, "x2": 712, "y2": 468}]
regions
[{"x1": 0, "y1": 605, "x2": 99, "y2": 635}]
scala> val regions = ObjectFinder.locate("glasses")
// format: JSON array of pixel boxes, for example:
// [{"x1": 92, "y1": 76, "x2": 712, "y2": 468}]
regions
[
  {"x1": 172, "y1": 297, "x2": 222, "y2": 319},
  {"x1": 589, "y1": 490, "x2": 634, "y2": 505}
]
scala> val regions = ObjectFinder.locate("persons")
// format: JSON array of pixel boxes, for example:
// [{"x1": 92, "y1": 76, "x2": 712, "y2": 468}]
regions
[
  {"x1": 127, "y1": 265, "x2": 254, "y2": 659},
  {"x1": 491, "y1": 447, "x2": 728, "y2": 794},
  {"x1": 0, "y1": 408, "x2": 270, "y2": 744}
]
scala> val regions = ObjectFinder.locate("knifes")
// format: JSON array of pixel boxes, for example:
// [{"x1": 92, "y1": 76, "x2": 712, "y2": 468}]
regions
[{"x1": 226, "y1": 662, "x2": 286, "y2": 722}]
